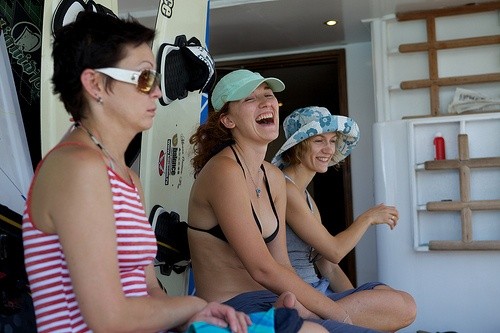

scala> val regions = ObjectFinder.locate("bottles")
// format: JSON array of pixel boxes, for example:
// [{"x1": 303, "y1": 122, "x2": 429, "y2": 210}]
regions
[{"x1": 433, "y1": 132, "x2": 445, "y2": 161}]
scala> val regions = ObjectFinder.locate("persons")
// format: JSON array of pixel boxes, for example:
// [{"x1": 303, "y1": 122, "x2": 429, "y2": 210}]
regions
[
  {"x1": 188, "y1": 70, "x2": 416, "y2": 333},
  {"x1": 273, "y1": 107, "x2": 400, "y2": 301},
  {"x1": 23, "y1": 11, "x2": 327, "y2": 333}
]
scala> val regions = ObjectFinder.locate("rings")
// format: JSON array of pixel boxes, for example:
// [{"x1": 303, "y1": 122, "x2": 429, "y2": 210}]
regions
[{"x1": 392, "y1": 216, "x2": 395, "y2": 220}]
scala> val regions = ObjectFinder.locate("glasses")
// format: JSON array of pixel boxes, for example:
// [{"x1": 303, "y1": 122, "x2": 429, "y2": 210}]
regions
[
  {"x1": 94, "y1": 67, "x2": 162, "y2": 95},
  {"x1": 309, "y1": 246, "x2": 320, "y2": 264}
]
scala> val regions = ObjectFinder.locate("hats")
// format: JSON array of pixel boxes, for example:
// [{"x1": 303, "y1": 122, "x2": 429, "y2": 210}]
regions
[
  {"x1": 271, "y1": 106, "x2": 360, "y2": 169},
  {"x1": 211, "y1": 69, "x2": 285, "y2": 113}
]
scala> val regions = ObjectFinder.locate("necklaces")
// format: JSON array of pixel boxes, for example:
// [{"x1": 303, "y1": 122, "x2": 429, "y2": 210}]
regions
[
  {"x1": 78, "y1": 126, "x2": 115, "y2": 161},
  {"x1": 236, "y1": 146, "x2": 261, "y2": 197}
]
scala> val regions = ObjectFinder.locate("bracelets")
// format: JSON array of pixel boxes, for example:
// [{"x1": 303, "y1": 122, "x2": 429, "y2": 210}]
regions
[{"x1": 344, "y1": 315, "x2": 350, "y2": 322}]
